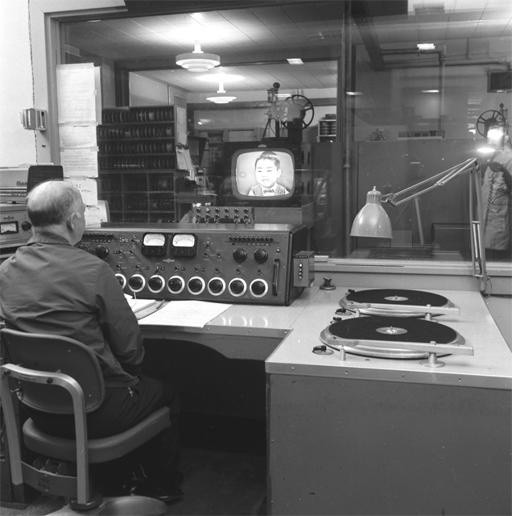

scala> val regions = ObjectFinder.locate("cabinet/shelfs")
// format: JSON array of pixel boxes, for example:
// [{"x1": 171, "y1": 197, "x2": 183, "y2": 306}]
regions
[{"x1": 97, "y1": 104, "x2": 182, "y2": 223}]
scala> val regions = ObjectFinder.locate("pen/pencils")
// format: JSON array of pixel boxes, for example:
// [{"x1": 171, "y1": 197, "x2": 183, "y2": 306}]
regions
[{"x1": 156, "y1": 299, "x2": 165, "y2": 309}]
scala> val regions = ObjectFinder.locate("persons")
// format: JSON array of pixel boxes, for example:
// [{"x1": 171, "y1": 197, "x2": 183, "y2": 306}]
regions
[
  {"x1": 247, "y1": 151, "x2": 289, "y2": 196},
  {"x1": 478, "y1": 121, "x2": 512, "y2": 260},
  {"x1": 0, "y1": 176, "x2": 188, "y2": 504}
]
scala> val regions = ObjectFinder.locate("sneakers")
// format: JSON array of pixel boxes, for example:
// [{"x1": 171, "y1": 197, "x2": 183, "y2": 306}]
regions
[{"x1": 122, "y1": 478, "x2": 185, "y2": 504}]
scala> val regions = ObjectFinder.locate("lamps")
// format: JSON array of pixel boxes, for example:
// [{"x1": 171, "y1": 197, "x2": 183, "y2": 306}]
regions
[{"x1": 348, "y1": 158, "x2": 497, "y2": 300}]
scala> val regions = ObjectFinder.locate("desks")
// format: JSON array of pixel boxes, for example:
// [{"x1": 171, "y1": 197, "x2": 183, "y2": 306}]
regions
[{"x1": 140, "y1": 285, "x2": 512, "y2": 516}]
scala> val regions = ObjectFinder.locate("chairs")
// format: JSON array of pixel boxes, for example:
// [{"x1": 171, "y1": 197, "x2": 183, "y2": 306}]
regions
[{"x1": 0, "y1": 322, "x2": 185, "y2": 516}]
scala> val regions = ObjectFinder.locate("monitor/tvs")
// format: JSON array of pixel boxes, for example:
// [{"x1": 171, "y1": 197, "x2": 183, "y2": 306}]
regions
[
  {"x1": 486, "y1": 69, "x2": 512, "y2": 93},
  {"x1": 231, "y1": 147, "x2": 296, "y2": 201}
]
